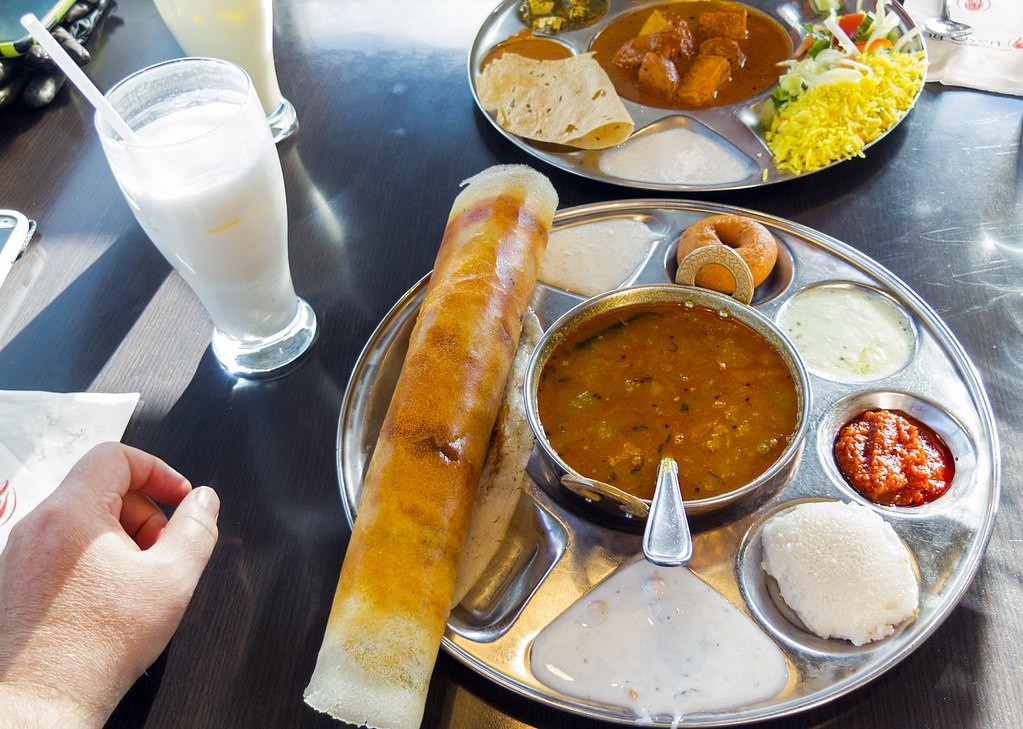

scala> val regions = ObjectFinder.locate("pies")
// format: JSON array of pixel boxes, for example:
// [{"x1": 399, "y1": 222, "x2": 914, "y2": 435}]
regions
[
  {"x1": 475, "y1": 51, "x2": 634, "y2": 149},
  {"x1": 302, "y1": 165, "x2": 560, "y2": 729}
]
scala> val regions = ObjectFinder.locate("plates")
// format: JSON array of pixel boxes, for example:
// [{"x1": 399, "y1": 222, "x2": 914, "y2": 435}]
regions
[
  {"x1": 465, "y1": 0, "x2": 933, "y2": 191},
  {"x1": 330, "y1": 198, "x2": 1005, "y2": 729}
]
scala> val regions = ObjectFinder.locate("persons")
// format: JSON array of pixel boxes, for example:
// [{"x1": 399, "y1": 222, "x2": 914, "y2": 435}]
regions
[{"x1": 0, "y1": 438, "x2": 224, "y2": 729}]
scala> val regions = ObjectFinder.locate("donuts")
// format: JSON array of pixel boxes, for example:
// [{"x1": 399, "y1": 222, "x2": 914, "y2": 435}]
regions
[{"x1": 676, "y1": 214, "x2": 779, "y2": 294}]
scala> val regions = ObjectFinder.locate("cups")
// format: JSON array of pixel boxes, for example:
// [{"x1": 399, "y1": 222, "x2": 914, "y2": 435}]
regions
[
  {"x1": 96, "y1": 56, "x2": 315, "y2": 379},
  {"x1": 150, "y1": 0, "x2": 301, "y2": 149}
]
